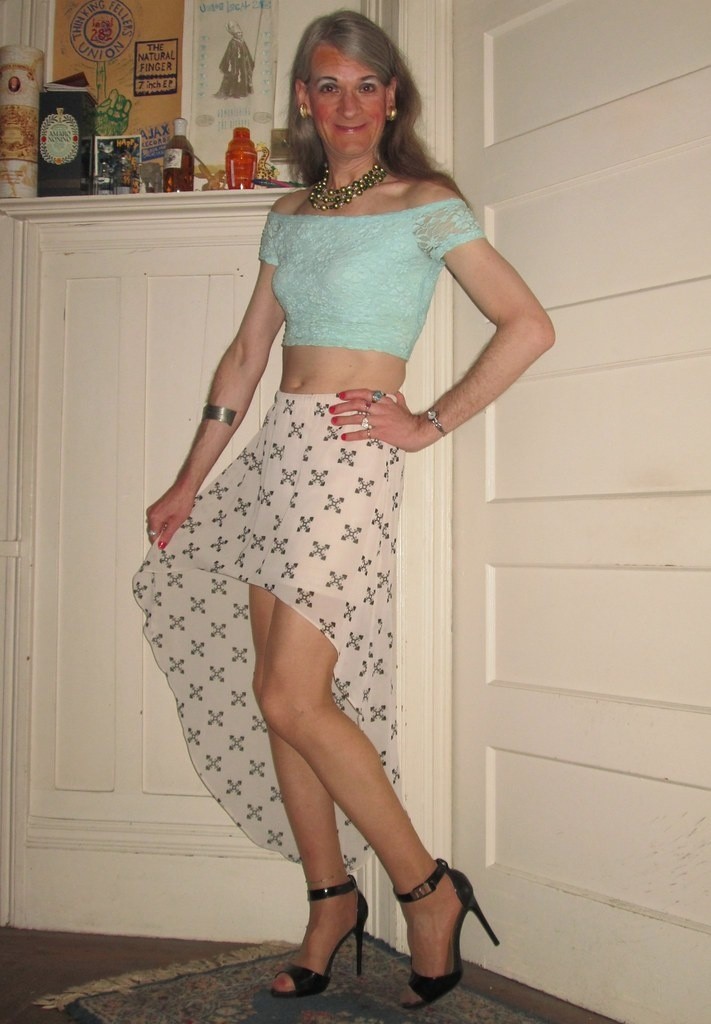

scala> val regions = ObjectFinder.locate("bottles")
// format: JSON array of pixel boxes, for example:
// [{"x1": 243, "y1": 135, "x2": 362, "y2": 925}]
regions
[
  {"x1": 163, "y1": 118, "x2": 194, "y2": 191},
  {"x1": 226, "y1": 127, "x2": 257, "y2": 189}
]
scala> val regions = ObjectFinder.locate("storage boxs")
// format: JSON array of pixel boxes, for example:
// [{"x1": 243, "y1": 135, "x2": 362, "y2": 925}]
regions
[{"x1": 37, "y1": 91, "x2": 98, "y2": 197}]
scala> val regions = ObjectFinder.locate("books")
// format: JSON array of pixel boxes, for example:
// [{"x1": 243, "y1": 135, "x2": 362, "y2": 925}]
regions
[{"x1": 42, "y1": 71, "x2": 98, "y2": 105}]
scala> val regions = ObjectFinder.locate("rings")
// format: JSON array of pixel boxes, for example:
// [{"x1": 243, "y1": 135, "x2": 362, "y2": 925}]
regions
[
  {"x1": 147, "y1": 530, "x2": 157, "y2": 536},
  {"x1": 361, "y1": 390, "x2": 384, "y2": 438}
]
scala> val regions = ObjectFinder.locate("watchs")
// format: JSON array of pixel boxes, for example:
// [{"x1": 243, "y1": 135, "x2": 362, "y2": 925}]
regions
[{"x1": 427, "y1": 407, "x2": 448, "y2": 437}]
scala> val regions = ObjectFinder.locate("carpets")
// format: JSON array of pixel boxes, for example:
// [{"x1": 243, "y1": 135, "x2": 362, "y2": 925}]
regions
[{"x1": 31, "y1": 929, "x2": 554, "y2": 1024}]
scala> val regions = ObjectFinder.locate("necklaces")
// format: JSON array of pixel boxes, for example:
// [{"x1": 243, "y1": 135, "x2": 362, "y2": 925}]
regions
[{"x1": 308, "y1": 164, "x2": 388, "y2": 211}]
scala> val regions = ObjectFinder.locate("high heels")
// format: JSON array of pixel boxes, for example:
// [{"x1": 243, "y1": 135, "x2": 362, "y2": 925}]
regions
[
  {"x1": 394, "y1": 858, "x2": 499, "y2": 1011},
  {"x1": 271, "y1": 874, "x2": 369, "y2": 999}
]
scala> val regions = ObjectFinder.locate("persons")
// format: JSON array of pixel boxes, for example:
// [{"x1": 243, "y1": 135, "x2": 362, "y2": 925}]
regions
[{"x1": 129, "y1": 9, "x2": 554, "y2": 1009}]
